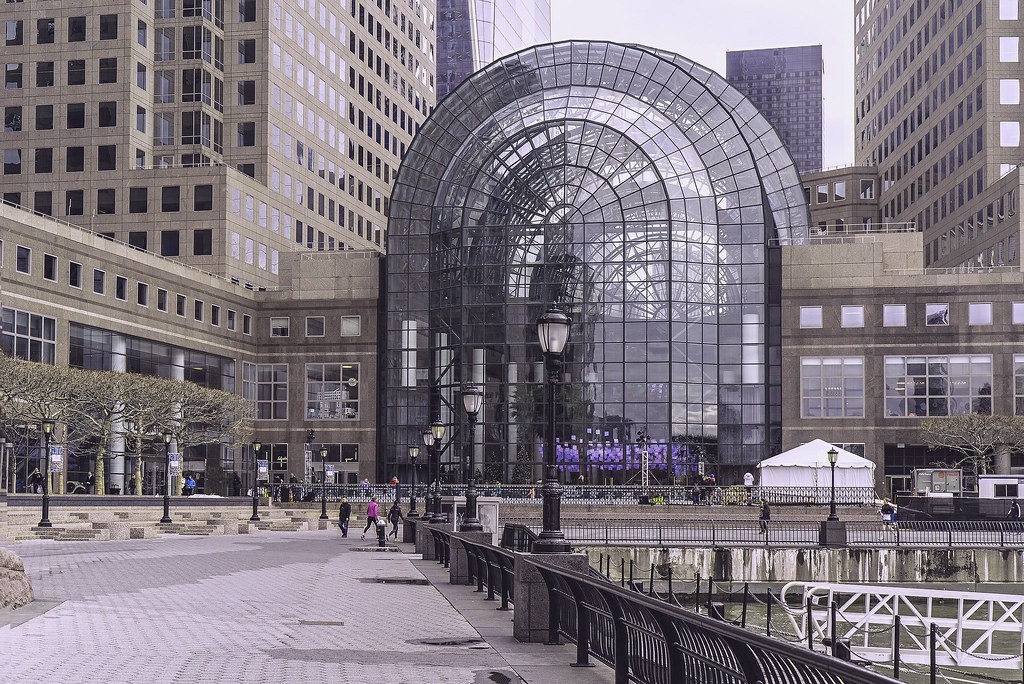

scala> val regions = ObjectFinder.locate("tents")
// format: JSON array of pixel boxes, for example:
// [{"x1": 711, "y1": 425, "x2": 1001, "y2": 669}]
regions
[{"x1": 755, "y1": 438, "x2": 876, "y2": 506}]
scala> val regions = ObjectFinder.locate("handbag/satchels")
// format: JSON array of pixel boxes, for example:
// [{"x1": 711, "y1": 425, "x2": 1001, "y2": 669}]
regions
[{"x1": 37, "y1": 486, "x2": 43, "y2": 493}]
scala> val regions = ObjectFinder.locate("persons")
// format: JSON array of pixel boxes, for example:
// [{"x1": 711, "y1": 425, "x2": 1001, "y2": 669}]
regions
[
  {"x1": 85, "y1": 471, "x2": 95, "y2": 494},
  {"x1": 703, "y1": 473, "x2": 716, "y2": 505},
  {"x1": 360, "y1": 478, "x2": 370, "y2": 490},
  {"x1": 128, "y1": 473, "x2": 136, "y2": 495},
  {"x1": 186, "y1": 475, "x2": 196, "y2": 489},
  {"x1": 289, "y1": 473, "x2": 298, "y2": 484},
  {"x1": 758, "y1": 501, "x2": 771, "y2": 534},
  {"x1": 743, "y1": 470, "x2": 755, "y2": 493},
  {"x1": 389, "y1": 474, "x2": 399, "y2": 495},
  {"x1": 1007, "y1": 499, "x2": 1021, "y2": 535},
  {"x1": 361, "y1": 496, "x2": 379, "y2": 541},
  {"x1": 233, "y1": 472, "x2": 242, "y2": 496},
  {"x1": 431, "y1": 478, "x2": 441, "y2": 487},
  {"x1": 338, "y1": 496, "x2": 351, "y2": 538},
  {"x1": 878, "y1": 499, "x2": 893, "y2": 530},
  {"x1": 29, "y1": 468, "x2": 43, "y2": 494},
  {"x1": 386, "y1": 501, "x2": 404, "y2": 542}
]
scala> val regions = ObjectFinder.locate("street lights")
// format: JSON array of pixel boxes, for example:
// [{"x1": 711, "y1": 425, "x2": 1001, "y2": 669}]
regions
[
  {"x1": 40, "y1": 416, "x2": 55, "y2": 526},
  {"x1": 429, "y1": 415, "x2": 446, "y2": 523},
  {"x1": 318, "y1": 445, "x2": 329, "y2": 519},
  {"x1": 458, "y1": 381, "x2": 484, "y2": 532},
  {"x1": 826, "y1": 447, "x2": 839, "y2": 521},
  {"x1": 408, "y1": 440, "x2": 419, "y2": 516},
  {"x1": 530, "y1": 301, "x2": 573, "y2": 555},
  {"x1": 250, "y1": 438, "x2": 262, "y2": 520},
  {"x1": 422, "y1": 425, "x2": 435, "y2": 520},
  {"x1": 160, "y1": 428, "x2": 172, "y2": 522}
]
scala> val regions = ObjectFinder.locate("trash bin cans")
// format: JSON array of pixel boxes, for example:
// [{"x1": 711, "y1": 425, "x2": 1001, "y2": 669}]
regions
[
  {"x1": 292, "y1": 487, "x2": 301, "y2": 502},
  {"x1": 109, "y1": 484, "x2": 121, "y2": 495},
  {"x1": 281, "y1": 486, "x2": 289, "y2": 502}
]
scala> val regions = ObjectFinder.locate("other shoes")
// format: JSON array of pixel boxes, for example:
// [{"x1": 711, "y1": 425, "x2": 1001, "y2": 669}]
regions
[
  {"x1": 386, "y1": 534, "x2": 390, "y2": 541},
  {"x1": 341, "y1": 533, "x2": 347, "y2": 538},
  {"x1": 394, "y1": 538, "x2": 400, "y2": 542},
  {"x1": 361, "y1": 535, "x2": 365, "y2": 541}
]
